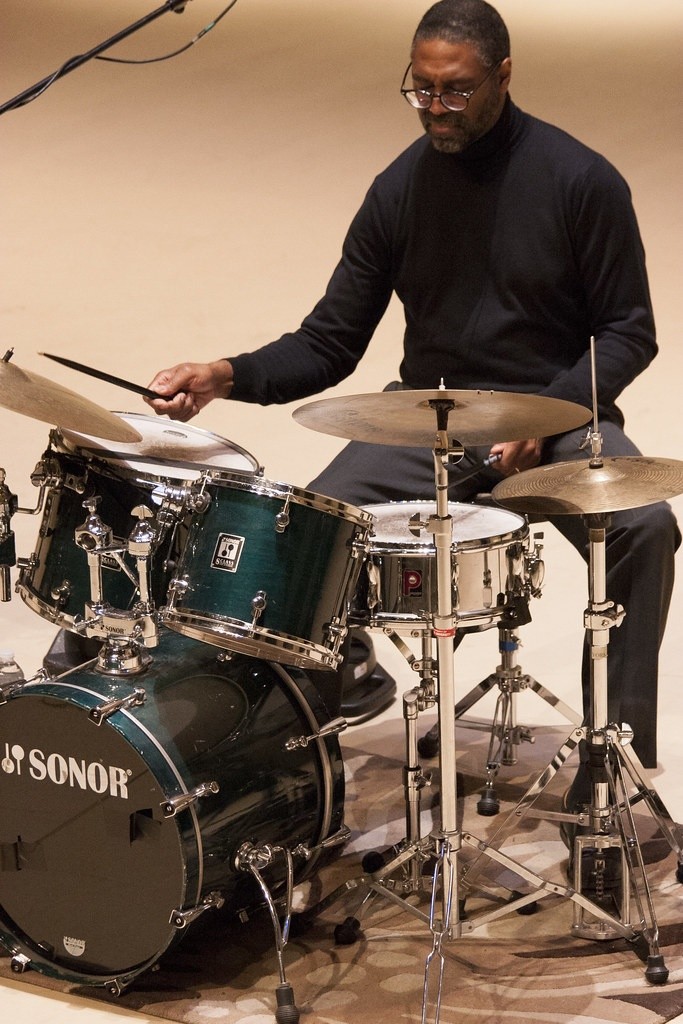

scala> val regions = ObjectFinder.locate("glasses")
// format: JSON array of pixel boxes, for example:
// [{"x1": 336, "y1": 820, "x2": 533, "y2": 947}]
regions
[{"x1": 400, "y1": 61, "x2": 500, "y2": 111}]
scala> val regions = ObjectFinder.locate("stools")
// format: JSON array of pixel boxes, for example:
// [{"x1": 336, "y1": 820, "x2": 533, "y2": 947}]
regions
[{"x1": 413, "y1": 481, "x2": 581, "y2": 815}]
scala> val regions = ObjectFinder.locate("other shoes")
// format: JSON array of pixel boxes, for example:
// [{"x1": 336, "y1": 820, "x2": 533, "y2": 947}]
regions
[{"x1": 559, "y1": 784, "x2": 621, "y2": 897}]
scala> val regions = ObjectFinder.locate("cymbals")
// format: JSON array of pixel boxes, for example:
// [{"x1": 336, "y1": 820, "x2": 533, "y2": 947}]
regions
[
  {"x1": 490, "y1": 456, "x2": 683, "y2": 516},
  {"x1": 291, "y1": 387, "x2": 595, "y2": 451},
  {"x1": 0, "y1": 357, "x2": 143, "y2": 443}
]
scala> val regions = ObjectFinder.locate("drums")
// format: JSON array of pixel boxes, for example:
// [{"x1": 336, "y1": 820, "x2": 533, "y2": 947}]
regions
[
  {"x1": 158, "y1": 469, "x2": 379, "y2": 674},
  {"x1": 0, "y1": 621, "x2": 348, "y2": 991},
  {"x1": 17, "y1": 410, "x2": 264, "y2": 642},
  {"x1": 337, "y1": 500, "x2": 534, "y2": 634}
]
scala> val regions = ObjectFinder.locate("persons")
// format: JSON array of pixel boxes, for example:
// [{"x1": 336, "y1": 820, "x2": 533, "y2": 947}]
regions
[{"x1": 141, "y1": 1, "x2": 683, "y2": 849}]
